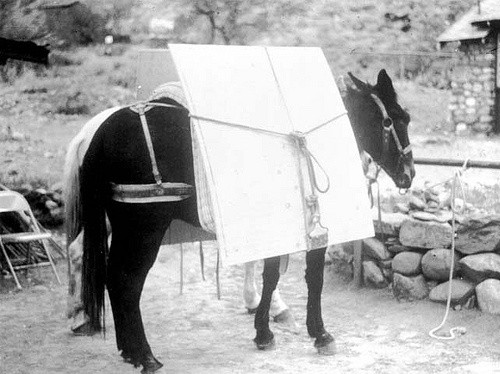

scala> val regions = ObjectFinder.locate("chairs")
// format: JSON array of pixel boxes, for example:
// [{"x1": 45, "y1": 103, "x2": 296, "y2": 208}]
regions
[{"x1": 0, "y1": 190, "x2": 62, "y2": 290}]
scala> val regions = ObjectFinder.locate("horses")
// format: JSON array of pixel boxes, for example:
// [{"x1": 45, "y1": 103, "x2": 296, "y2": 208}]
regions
[{"x1": 64, "y1": 69, "x2": 416, "y2": 374}]
